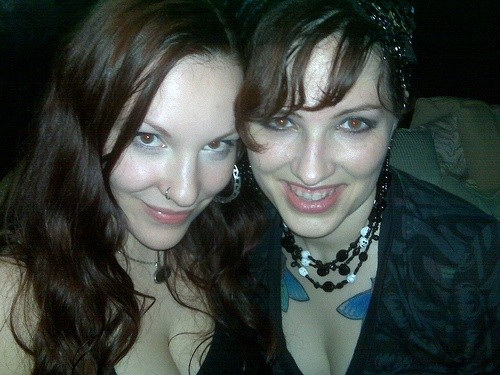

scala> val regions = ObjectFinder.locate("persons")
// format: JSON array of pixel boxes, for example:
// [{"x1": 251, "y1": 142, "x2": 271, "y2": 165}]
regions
[
  {"x1": 238, "y1": 0, "x2": 499, "y2": 375},
  {"x1": 0, "y1": 0, "x2": 244, "y2": 375}
]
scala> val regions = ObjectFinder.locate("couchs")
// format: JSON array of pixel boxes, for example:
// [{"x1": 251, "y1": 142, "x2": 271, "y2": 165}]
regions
[{"x1": 389, "y1": 93, "x2": 500, "y2": 222}]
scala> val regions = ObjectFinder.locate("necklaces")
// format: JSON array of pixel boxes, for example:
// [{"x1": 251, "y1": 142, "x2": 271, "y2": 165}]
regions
[
  {"x1": 114, "y1": 242, "x2": 174, "y2": 285},
  {"x1": 276, "y1": 168, "x2": 384, "y2": 290}
]
